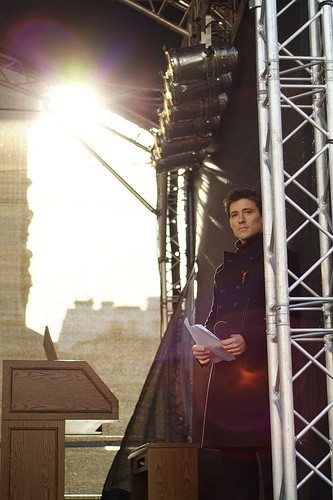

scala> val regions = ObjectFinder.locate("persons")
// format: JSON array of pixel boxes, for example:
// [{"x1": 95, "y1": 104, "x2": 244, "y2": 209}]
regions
[{"x1": 184, "y1": 188, "x2": 298, "y2": 500}]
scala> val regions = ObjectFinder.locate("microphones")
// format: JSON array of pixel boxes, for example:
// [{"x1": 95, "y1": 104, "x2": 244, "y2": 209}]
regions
[{"x1": 214, "y1": 321, "x2": 244, "y2": 360}]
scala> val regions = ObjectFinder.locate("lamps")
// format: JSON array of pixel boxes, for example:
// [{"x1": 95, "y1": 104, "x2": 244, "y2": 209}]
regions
[{"x1": 151, "y1": 44, "x2": 238, "y2": 173}]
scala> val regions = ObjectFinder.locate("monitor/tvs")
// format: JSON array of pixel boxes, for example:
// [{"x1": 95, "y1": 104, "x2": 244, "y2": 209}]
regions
[{"x1": 42, "y1": 326, "x2": 59, "y2": 361}]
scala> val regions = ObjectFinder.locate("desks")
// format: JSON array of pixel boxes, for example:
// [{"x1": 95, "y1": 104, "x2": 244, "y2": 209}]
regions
[{"x1": 131, "y1": 443, "x2": 200, "y2": 500}]
